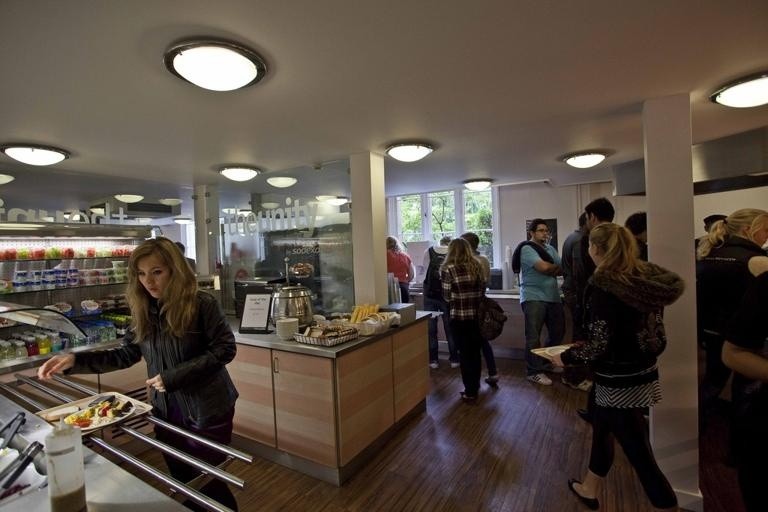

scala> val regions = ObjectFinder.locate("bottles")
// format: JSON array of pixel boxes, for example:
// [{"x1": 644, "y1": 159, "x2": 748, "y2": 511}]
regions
[
  {"x1": 0, "y1": 329, "x2": 62, "y2": 359},
  {"x1": 100, "y1": 312, "x2": 132, "y2": 338},
  {"x1": 45, "y1": 415, "x2": 88, "y2": 512},
  {"x1": 65, "y1": 319, "x2": 117, "y2": 347}
]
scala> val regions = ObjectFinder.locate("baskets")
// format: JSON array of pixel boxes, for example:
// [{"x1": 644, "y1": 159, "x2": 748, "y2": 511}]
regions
[
  {"x1": 292, "y1": 332, "x2": 358, "y2": 346},
  {"x1": 343, "y1": 321, "x2": 391, "y2": 336}
]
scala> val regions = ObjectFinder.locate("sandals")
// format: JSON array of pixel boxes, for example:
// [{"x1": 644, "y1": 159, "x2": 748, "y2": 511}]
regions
[{"x1": 568, "y1": 477, "x2": 600, "y2": 510}]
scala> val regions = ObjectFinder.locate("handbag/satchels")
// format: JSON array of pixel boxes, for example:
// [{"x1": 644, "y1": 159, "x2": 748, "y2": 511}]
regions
[{"x1": 473, "y1": 296, "x2": 508, "y2": 341}]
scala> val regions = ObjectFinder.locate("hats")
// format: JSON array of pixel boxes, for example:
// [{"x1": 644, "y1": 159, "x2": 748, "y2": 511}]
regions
[{"x1": 704, "y1": 214, "x2": 728, "y2": 224}]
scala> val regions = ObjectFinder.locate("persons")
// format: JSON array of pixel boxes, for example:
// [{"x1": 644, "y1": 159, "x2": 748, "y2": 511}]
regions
[
  {"x1": 440, "y1": 239, "x2": 486, "y2": 401},
  {"x1": 579, "y1": 197, "x2": 615, "y2": 277},
  {"x1": 625, "y1": 213, "x2": 649, "y2": 264},
  {"x1": 461, "y1": 232, "x2": 499, "y2": 383},
  {"x1": 694, "y1": 214, "x2": 729, "y2": 253},
  {"x1": 721, "y1": 271, "x2": 767, "y2": 511},
  {"x1": 421, "y1": 236, "x2": 461, "y2": 369},
  {"x1": 551, "y1": 222, "x2": 682, "y2": 512},
  {"x1": 516, "y1": 219, "x2": 566, "y2": 386},
  {"x1": 173, "y1": 240, "x2": 195, "y2": 275},
  {"x1": 563, "y1": 213, "x2": 598, "y2": 390},
  {"x1": 37, "y1": 236, "x2": 240, "y2": 511},
  {"x1": 385, "y1": 236, "x2": 415, "y2": 304},
  {"x1": 695, "y1": 208, "x2": 768, "y2": 419}
]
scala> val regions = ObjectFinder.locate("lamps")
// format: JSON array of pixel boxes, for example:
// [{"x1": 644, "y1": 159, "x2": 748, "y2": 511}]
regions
[
  {"x1": 1, "y1": 145, "x2": 70, "y2": 166},
  {"x1": 159, "y1": 198, "x2": 183, "y2": 207},
  {"x1": 262, "y1": 202, "x2": 281, "y2": 209},
  {"x1": 562, "y1": 151, "x2": 608, "y2": 169},
  {"x1": 163, "y1": 40, "x2": 266, "y2": 93},
  {"x1": 709, "y1": 71, "x2": 768, "y2": 109},
  {"x1": 384, "y1": 143, "x2": 436, "y2": 163},
  {"x1": 112, "y1": 193, "x2": 144, "y2": 203},
  {"x1": 462, "y1": 178, "x2": 494, "y2": 191},
  {"x1": 218, "y1": 166, "x2": 261, "y2": 182},
  {"x1": 265, "y1": 177, "x2": 297, "y2": 189},
  {"x1": 0, "y1": 173, "x2": 14, "y2": 186}
]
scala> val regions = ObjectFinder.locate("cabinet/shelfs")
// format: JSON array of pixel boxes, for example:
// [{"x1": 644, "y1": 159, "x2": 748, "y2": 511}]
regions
[
  {"x1": 0, "y1": 222, "x2": 164, "y2": 375},
  {"x1": 271, "y1": 350, "x2": 338, "y2": 469},
  {"x1": 225, "y1": 344, "x2": 277, "y2": 449}
]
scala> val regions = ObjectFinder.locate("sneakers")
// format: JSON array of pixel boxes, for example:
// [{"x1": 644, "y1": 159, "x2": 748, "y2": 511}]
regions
[
  {"x1": 458, "y1": 391, "x2": 477, "y2": 401},
  {"x1": 485, "y1": 377, "x2": 499, "y2": 383},
  {"x1": 430, "y1": 363, "x2": 439, "y2": 368},
  {"x1": 450, "y1": 362, "x2": 460, "y2": 368},
  {"x1": 560, "y1": 375, "x2": 592, "y2": 391},
  {"x1": 526, "y1": 373, "x2": 552, "y2": 386}
]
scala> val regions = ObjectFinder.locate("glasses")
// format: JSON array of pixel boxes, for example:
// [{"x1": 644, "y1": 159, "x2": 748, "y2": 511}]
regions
[{"x1": 537, "y1": 228, "x2": 549, "y2": 233}]
